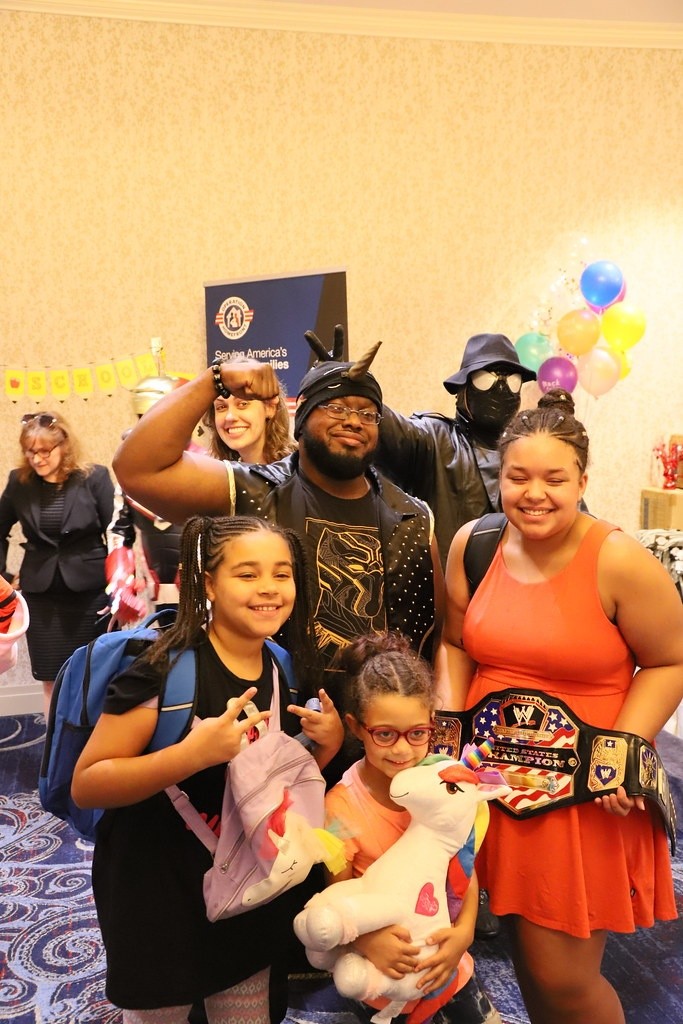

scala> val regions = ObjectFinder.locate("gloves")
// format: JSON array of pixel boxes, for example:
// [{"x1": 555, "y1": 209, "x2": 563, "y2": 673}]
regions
[{"x1": 303, "y1": 324, "x2": 343, "y2": 373}]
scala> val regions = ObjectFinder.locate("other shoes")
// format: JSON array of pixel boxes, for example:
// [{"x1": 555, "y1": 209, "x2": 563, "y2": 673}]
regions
[{"x1": 476, "y1": 890, "x2": 500, "y2": 936}]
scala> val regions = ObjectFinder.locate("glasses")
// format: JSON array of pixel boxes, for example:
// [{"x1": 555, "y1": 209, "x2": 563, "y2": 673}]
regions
[
  {"x1": 24, "y1": 442, "x2": 62, "y2": 458},
  {"x1": 470, "y1": 369, "x2": 523, "y2": 394},
  {"x1": 361, "y1": 722, "x2": 435, "y2": 747},
  {"x1": 318, "y1": 404, "x2": 384, "y2": 425},
  {"x1": 21, "y1": 414, "x2": 58, "y2": 428}
]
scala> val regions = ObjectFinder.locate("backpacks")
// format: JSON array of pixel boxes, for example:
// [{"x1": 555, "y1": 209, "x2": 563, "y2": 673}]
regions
[
  {"x1": 164, "y1": 659, "x2": 348, "y2": 923},
  {"x1": 38, "y1": 609, "x2": 300, "y2": 844}
]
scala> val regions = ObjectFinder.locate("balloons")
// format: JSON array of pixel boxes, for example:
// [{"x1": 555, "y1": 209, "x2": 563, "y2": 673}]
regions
[{"x1": 512, "y1": 259, "x2": 646, "y2": 405}]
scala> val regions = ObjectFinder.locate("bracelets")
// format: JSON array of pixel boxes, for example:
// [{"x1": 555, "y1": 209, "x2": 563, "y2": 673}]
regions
[{"x1": 212, "y1": 356, "x2": 234, "y2": 404}]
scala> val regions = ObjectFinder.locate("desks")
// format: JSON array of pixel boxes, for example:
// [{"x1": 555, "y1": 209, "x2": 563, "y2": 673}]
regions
[{"x1": 640, "y1": 486, "x2": 683, "y2": 533}]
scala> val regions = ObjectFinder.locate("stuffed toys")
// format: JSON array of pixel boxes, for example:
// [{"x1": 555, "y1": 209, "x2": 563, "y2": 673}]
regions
[{"x1": 293, "y1": 739, "x2": 552, "y2": 1019}]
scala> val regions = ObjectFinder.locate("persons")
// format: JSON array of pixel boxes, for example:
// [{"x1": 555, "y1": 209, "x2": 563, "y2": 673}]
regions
[
  {"x1": 2, "y1": 412, "x2": 115, "y2": 736},
  {"x1": 200, "y1": 346, "x2": 294, "y2": 466},
  {"x1": 429, "y1": 393, "x2": 683, "y2": 1022},
  {"x1": 124, "y1": 371, "x2": 196, "y2": 599},
  {"x1": 323, "y1": 634, "x2": 506, "y2": 1023},
  {"x1": 71, "y1": 514, "x2": 342, "y2": 1024},
  {"x1": 110, "y1": 321, "x2": 442, "y2": 744},
  {"x1": 302, "y1": 310, "x2": 536, "y2": 573}
]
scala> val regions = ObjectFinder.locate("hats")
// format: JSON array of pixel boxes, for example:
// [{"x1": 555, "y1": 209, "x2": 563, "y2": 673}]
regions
[
  {"x1": 443, "y1": 332, "x2": 537, "y2": 395},
  {"x1": 294, "y1": 341, "x2": 382, "y2": 442}
]
